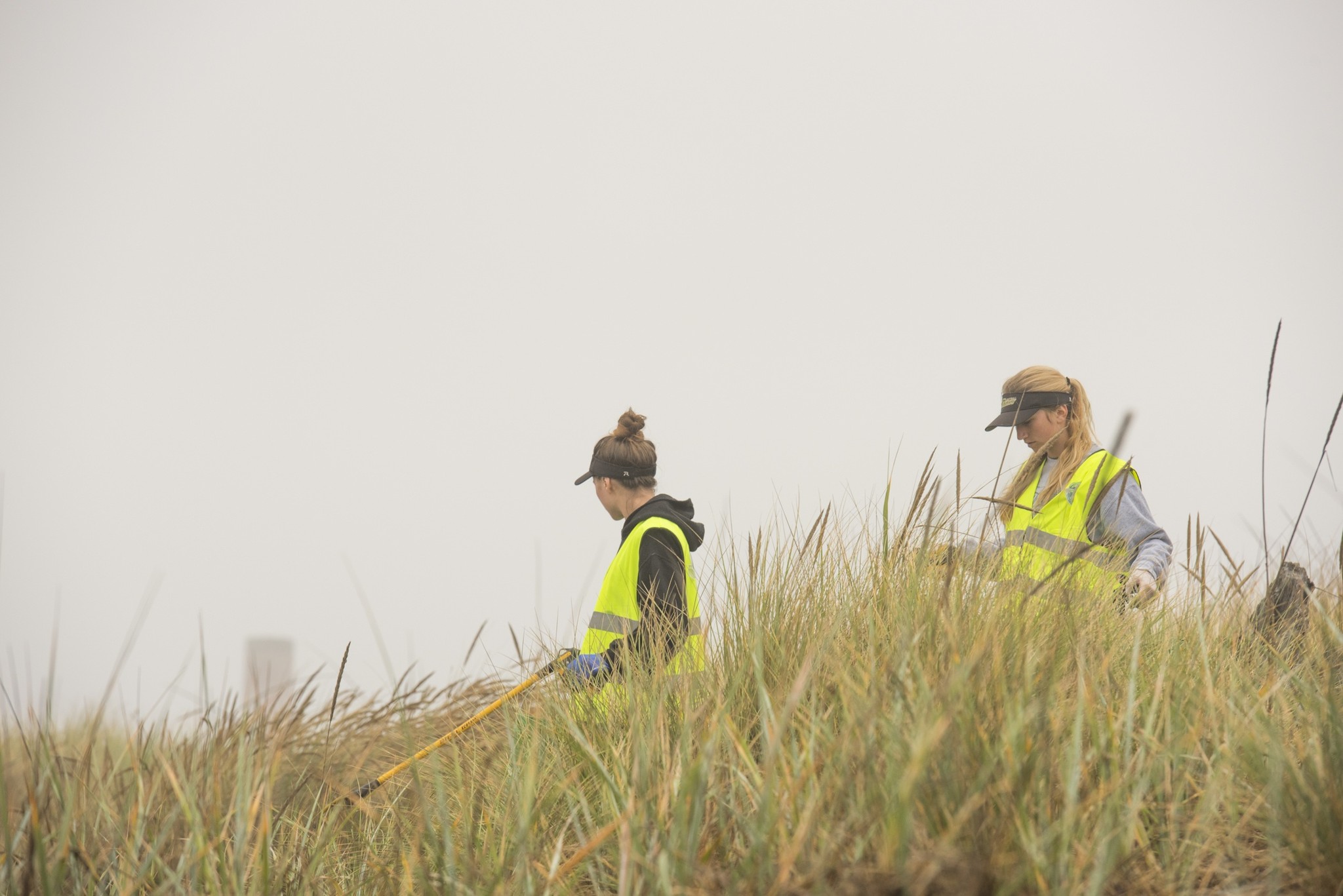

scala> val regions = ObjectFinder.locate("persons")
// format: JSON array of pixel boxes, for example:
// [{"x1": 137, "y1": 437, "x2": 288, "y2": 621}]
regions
[
  {"x1": 918, "y1": 365, "x2": 1173, "y2": 612},
  {"x1": 556, "y1": 408, "x2": 709, "y2": 724}
]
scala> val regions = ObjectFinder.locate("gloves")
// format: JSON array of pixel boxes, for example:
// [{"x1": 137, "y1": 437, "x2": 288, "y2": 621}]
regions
[{"x1": 565, "y1": 652, "x2": 609, "y2": 692}]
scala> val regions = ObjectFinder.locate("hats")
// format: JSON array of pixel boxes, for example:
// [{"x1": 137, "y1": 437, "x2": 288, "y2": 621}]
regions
[
  {"x1": 575, "y1": 454, "x2": 656, "y2": 486},
  {"x1": 984, "y1": 392, "x2": 1070, "y2": 432}
]
